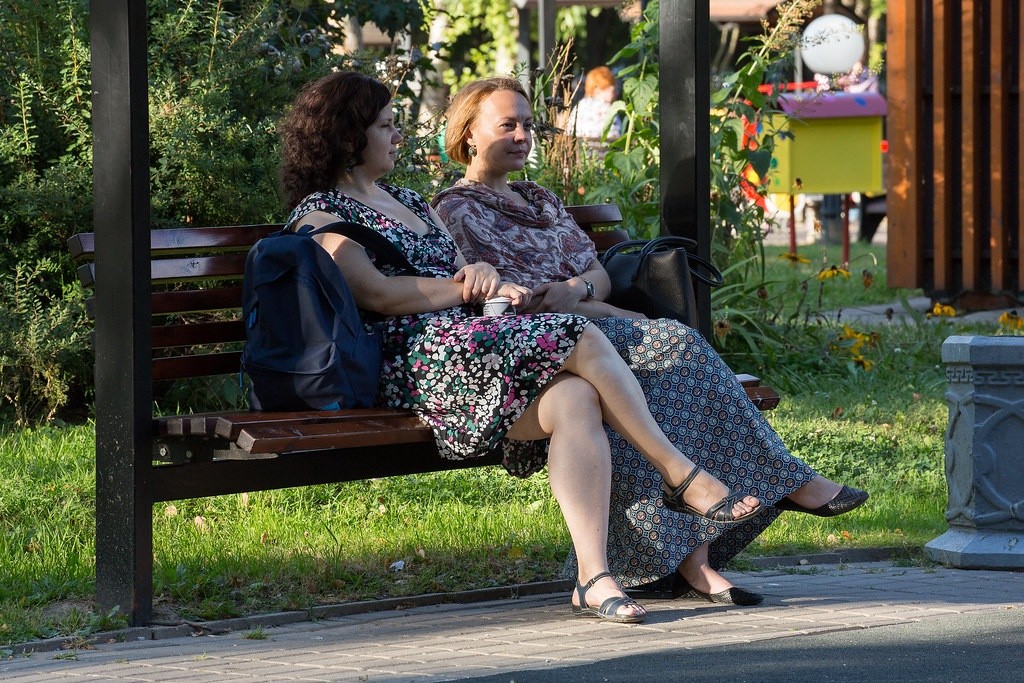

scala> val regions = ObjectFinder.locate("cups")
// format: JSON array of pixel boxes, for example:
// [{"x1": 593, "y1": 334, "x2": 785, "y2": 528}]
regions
[{"x1": 481, "y1": 295, "x2": 516, "y2": 316}]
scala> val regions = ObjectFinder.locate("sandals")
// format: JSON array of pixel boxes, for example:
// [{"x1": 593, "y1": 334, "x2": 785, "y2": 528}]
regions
[
  {"x1": 571, "y1": 571, "x2": 648, "y2": 623},
  {"x1": 662, "y1": 465, "x2": 765, "y2": 524}
]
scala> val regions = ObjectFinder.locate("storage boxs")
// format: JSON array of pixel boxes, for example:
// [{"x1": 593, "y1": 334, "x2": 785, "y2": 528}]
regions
[{"x1": 745, "y1": 89, "x2": 888, "y2": 194}]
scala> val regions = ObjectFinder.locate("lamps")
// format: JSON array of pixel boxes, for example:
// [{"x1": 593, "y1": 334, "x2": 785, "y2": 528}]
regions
[{"x1": 801, "y1": 15, "x2": 865, "y2": 92}]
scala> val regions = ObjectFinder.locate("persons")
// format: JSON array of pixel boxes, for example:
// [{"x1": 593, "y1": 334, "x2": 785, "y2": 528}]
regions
[
  {"x1": 279, "y1": 73, "x2": 763, "y2": 623},
  {"x1": 430, "y1": 78, "x2": 868, "y2": 605},
  {"x1": 567, "y1": 67, "x2": 623, "y2": 163}
]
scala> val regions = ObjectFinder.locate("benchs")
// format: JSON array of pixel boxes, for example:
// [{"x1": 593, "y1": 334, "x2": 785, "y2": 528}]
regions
[{"x1": 67, "y1": 203, "x2": 781, "y2": 508}]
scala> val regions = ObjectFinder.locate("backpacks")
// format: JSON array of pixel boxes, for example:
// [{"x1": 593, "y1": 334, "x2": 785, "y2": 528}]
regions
[{"x1": 238, "y1": 221, "x2": 420, "y2": 412}]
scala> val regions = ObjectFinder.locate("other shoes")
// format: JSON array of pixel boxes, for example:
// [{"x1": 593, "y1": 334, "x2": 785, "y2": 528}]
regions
[
  {"x1": 774, "y1": 485, "x2": 870, "y2": 517},
  {"x1": 670, "y1": 569, "x2": 765, "y2": 606}
]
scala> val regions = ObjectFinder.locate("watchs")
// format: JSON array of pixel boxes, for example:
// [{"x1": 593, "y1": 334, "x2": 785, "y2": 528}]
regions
[{"x1": 579, "y1": 276, "x2": 595, "y2": 301}]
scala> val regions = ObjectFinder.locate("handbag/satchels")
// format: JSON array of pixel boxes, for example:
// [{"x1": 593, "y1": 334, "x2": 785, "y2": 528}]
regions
[{"x1": 600, "y1": 236, "x2": 724, "y2": 331}]
